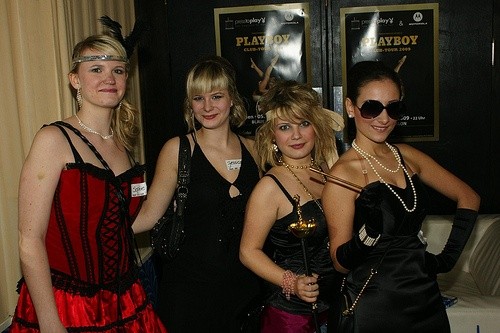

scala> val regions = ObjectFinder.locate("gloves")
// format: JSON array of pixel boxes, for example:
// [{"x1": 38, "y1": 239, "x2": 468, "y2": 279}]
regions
[
  {"x1": 336, "y1": 189, "x2": 386, "y2": 269},
  {"x1": 425, "y1": 207, "x2": 477, "y2": 275}
]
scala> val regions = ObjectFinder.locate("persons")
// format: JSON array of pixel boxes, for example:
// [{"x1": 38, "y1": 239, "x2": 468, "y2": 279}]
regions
[
  {"x1": 395, "y1": 55, "x2": 407, "y2": 73},
  {"x1": 248, "y1": 55, "x2": 281, "y2": 137},
  {"x1": 321, "y1": 61, "x2": 482, "y2": 333},
  {"x1": 9, "y1": 16, "x2": 165, "y2": 333},
  {"x1": 238, "y1": 81, "x2": 342, "y2": 333},
  {"x1": 131, "y1": 55, "x2": 276, "y2": 333}
]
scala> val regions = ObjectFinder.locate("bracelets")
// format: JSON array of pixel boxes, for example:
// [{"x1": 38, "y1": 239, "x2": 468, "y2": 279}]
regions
[
  {"x1": 281, "y1": 270, "x2": 296, "y2": 299},
  {"x1": 359, "y1": 224, "x2": 381, "y2": 247}
]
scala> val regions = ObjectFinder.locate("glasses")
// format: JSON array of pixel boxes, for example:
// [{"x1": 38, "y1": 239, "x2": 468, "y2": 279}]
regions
[{"x1": 354, "y1": 99, "x2": 407, "y2": 121}]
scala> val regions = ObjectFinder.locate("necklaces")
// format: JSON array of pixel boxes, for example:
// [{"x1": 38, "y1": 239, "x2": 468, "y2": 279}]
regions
[
  {"x1": 351, "y1": 137, "x2": 417, "y2": 213},
  {"x1": 280, "y1": 159, "x2": 313, "y2": 169},
  {"x1": 74, "y1": 114, "x2": 113, "y2": 140},
  {"x1": 287, "y1": 167, "x2": 326, "y2": 214}
]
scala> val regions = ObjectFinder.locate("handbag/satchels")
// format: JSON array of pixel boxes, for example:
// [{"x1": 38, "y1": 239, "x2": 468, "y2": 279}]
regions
[
  {"x1": 148, "y1": 136, "x2": 192, "y2": 261},
  {"x1": 142, "y1": 251, "x2": 169, "y2": 287},
  {"x1": 329, "y1": 313, "x2": 354, "y2": 333},
  {"x1": 239, "y1": 309, "x2": 259, "y2": 333}
]
